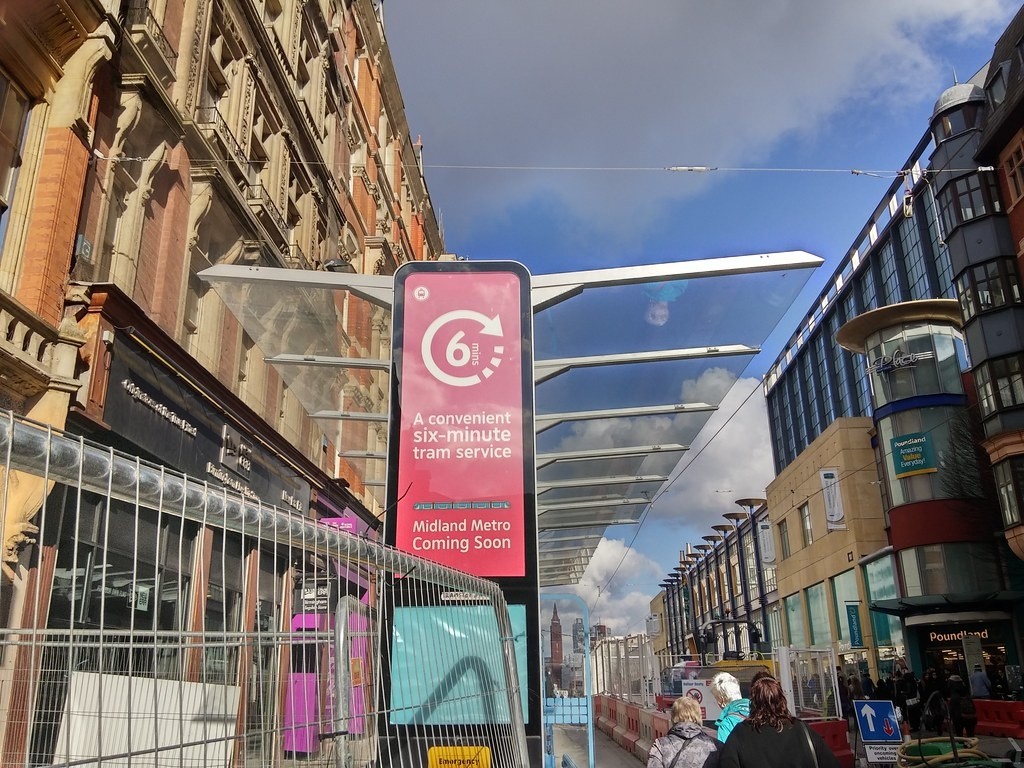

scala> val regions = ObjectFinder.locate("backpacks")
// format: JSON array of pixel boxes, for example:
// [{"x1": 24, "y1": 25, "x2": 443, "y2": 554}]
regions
[{"x1": 959, "y1": 693, "x2": 976, "y2": 719}]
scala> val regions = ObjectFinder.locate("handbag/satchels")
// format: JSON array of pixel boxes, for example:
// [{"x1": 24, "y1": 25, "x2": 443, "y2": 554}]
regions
[
  {"x1": 906, "y1": 698, "x2": 920, "y2": 706},
  {"x1": 895, "y1": 705, "x2": 903, "y2": 723},
  {"x1": 924, "y1": 705, "x2": 934, "y2": 723}
]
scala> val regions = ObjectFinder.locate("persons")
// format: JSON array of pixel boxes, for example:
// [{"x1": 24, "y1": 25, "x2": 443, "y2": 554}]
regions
[
  {"x1": 646, "y1": 671, "x2": 841, "y2": 768},
  {"x1": 792, "y1": 663, "x2": 1024, "y2": 747}
]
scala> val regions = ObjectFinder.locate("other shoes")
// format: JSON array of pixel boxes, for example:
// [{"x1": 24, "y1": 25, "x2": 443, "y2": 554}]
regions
[{"x1": 909, "y1": 726, "x2": 920, "y2": 733}]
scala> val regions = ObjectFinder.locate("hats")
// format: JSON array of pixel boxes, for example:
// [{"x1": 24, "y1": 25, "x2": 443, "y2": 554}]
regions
[
  {"x1": 973, "y1": 664, "x2": 984, "y2": 670},
  {"x1": 950, "y1": 675, "x2": 962, "y2": 682}
]
